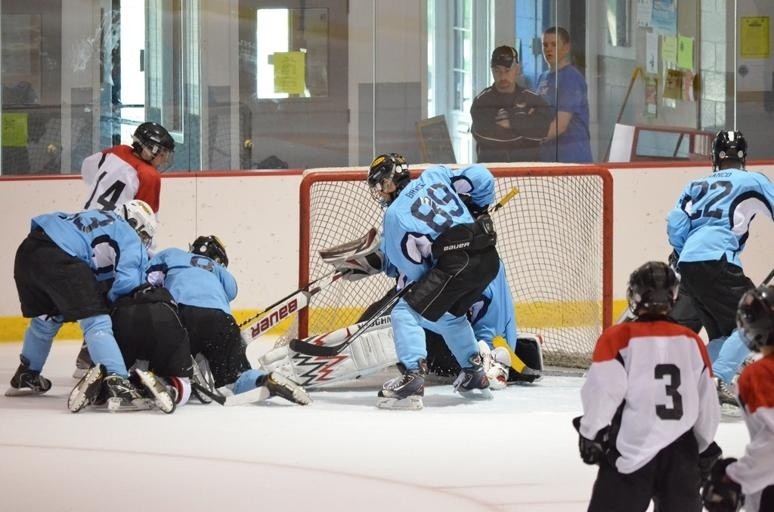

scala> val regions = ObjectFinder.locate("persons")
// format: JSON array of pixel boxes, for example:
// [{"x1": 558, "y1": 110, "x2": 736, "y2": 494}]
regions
[
  {"x1": 5, "y1": 200, "x2": 157, "y2": 410},
  {"x1": 68, "y1": 235, "x2": 313, "y2": 412},
  {"x1": 705, "y1": 283, "x2": 774, "y2": 512},
  {"x1": 574, "y1": 259, "x2": 709, "y2": 511},
  {"x1": 663, "y1": 127, "x2": 774, "y2": 344},
  {"x1": 533, "y1": 26, "x2": 595, "y2": 165},
  {"x1": 259, "y1": 228, "x2": 517, "y2": 389},
  {"x1": 367, "y1": 154, "x2": 529, "y2": 409},
  {"x1": 73, "y1": 121, "x2": 175, "y2": 379},
  {"x1": 469, "y1": 45, "x2": 550, "y2": 163}
]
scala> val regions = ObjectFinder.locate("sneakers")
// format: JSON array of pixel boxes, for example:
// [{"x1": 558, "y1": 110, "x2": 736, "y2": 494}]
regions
[
  {"x1": 263, "y1": 370, "x2": 310, "y2": 404},
  {"x1": 10, "y1": 340, "x2": 179, "y2": 414}
]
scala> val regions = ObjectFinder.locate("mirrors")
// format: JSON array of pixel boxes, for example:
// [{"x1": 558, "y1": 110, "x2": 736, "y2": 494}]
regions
[{"x1": 256, "y1": 6, "x2": 330, "y2": 101}]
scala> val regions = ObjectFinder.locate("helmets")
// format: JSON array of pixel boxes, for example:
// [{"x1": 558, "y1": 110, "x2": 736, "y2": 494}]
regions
[
  {"x1": 114, "y1": 199, "x2": 157, "y2": 238},
  {"x1": 626, "y1": 262, "x2": 678, "y2": 314},
  {"x1": 132, "y1": 122, "x2": 176, "y2": 152},
  {"x1": 368, "y1": 152, "x2": 409, "y2": 193},
  {"x1": 736, "y1": 287, "x2": 774, "y2": 352},
  {"x1": 712, "y1": 129, "x2": 748, "y2": 165},
  {"x1": 188, "y1": 235, "x2": 228, "y2": 266}
]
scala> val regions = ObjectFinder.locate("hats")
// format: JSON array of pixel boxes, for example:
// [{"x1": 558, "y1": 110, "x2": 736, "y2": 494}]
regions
[{"x1": 492, "y1": 46, "x2": 518, "y2": 68}]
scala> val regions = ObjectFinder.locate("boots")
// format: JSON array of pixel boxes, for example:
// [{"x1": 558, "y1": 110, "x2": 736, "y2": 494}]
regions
[
  {"x1": 454, "y1": 352, "x2": 490, "y2": 392},
  {"x1": 378, "y1": 358, "x2": 428, "y2": 397}
]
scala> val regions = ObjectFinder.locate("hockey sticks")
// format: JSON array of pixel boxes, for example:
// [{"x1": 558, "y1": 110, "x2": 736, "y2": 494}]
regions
[
  {"x1": 288, "y1": 186, "x2": 518, "y2": 355},
  {"x1": 191, "y1": 379, "x2": 270, "y2": 407}
]
